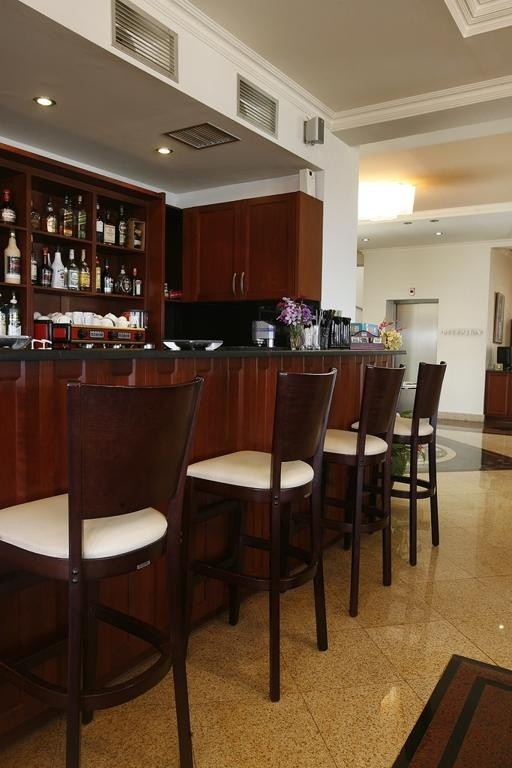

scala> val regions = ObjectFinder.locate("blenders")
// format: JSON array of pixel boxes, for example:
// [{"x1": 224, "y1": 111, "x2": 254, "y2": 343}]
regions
[{"x1": 250, "y1": 319, "x2": 275, "y2": 348}]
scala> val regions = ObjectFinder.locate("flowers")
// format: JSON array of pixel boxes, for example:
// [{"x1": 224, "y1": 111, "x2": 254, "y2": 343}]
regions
[{"x1": 276, "y1": 296, "x2": 312, "y2": 346}]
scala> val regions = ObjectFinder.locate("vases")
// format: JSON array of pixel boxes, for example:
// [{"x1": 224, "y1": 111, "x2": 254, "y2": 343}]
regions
[{"x1": 289, "y1": 329, "x2": 305, "y2": 351}]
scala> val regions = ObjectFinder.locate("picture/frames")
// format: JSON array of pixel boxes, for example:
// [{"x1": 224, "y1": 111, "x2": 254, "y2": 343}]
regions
[{"x1": 493, "y1": 292, "x2": 505, "y2": 344}]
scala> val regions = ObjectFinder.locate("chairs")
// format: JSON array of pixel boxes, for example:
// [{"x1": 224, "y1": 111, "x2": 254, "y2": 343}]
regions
[
  {"x1": 308, "y1": 363, "x2": 407, "y2": 616},
  {"x1": 344, "y1": 360, "x2": 447, "y2": 567},
  {"x1": 167, "y1": 366, "x2": 338, "y2": 702},
  {"x1": 0, "y1": 375, "x2": 207, "y2": 768}
]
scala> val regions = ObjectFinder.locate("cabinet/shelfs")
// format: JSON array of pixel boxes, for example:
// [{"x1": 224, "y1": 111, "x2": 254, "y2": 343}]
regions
[
  {"x1": 181, "y1": 191, "x2": 323, "y2": 304},
  {"x1": 485, "y1": 369, "x2": 512, "y2": 428},
  {"x1": 0, "y1": 141, "x2": 166, "y2": 351}
]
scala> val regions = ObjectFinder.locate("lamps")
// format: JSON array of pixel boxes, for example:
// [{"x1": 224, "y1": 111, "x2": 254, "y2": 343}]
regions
[{"x1": 357, "y1": 181, "x2": 416, "y2": 223}]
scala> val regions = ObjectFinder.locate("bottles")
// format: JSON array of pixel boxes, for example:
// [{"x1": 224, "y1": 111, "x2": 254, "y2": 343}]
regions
[{"x1": 0, "y1": 187, "x2": 145, "y2": 337}]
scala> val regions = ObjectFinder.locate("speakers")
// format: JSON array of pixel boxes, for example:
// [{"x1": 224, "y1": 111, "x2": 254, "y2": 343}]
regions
[{"x1": 305, "y1": 116, "x2": 324, "y2": 145}]
